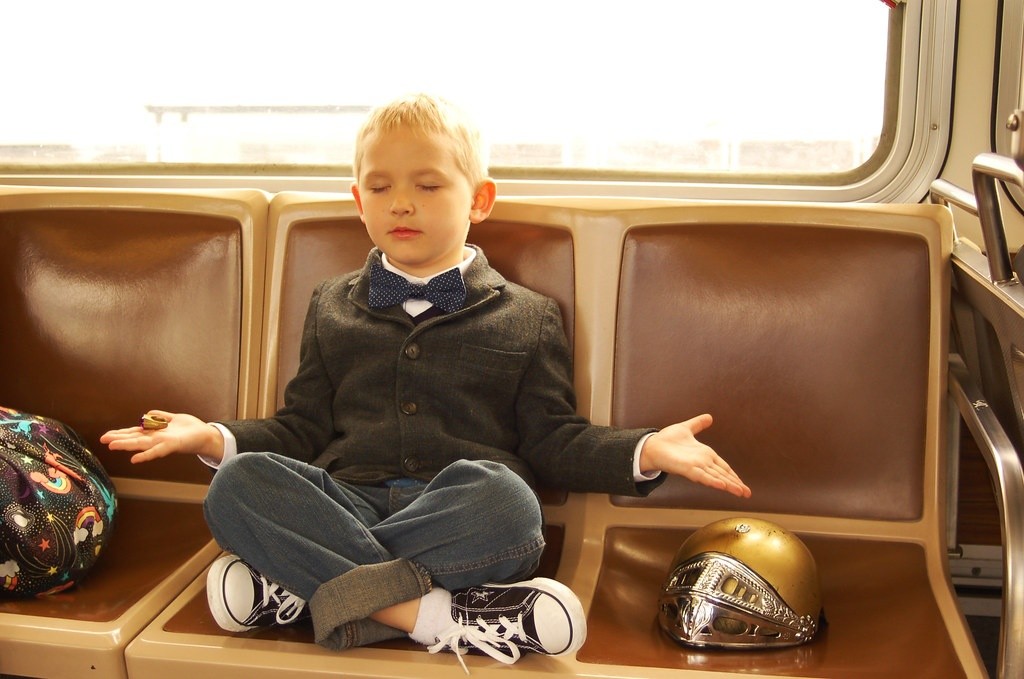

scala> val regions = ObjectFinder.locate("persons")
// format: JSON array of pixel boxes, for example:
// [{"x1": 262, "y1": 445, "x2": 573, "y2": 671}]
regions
[{"x1": 101, "y1": 97, "x2": 753, "y2": 665}]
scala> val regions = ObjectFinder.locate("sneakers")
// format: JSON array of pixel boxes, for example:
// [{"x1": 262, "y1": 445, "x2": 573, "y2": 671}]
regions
[
  {"x1": 204, "y1": 554, "x2": 307, "y2": 633},
  {"x1": 426, "y1": 576, "x2": 588, "y2": 673}
]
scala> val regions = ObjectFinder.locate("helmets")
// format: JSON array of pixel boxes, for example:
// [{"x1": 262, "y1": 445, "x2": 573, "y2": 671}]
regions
[{"x1": 656, "y1": 518, "x2": 822, "y2": 648}]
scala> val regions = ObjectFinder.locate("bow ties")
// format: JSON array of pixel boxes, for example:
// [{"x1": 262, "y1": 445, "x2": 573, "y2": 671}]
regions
[{"x1": 367, "y1": 263, "x2": 467, "y2": 311}]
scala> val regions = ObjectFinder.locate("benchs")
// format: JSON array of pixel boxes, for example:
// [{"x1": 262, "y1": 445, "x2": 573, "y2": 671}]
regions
[
  {"x1": 0, "y1": 188, "x2": 1024, "y2": 679},
  {"x1": 930, "y1": 150, "x2": 1024, "y2": 459}
]
